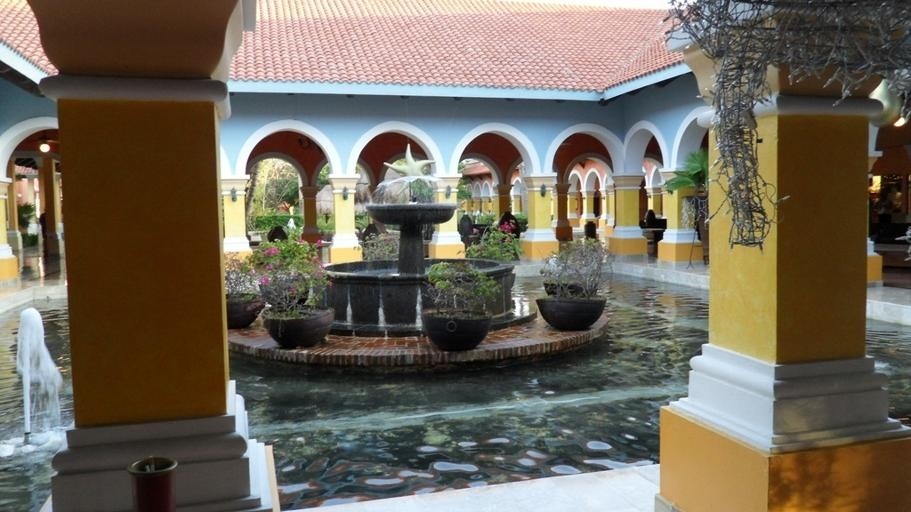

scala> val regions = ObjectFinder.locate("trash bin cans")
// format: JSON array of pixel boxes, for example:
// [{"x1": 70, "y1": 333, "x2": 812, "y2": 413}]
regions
[{"x1": 127, "y1": 455, "x2": 180, "y2": 512}]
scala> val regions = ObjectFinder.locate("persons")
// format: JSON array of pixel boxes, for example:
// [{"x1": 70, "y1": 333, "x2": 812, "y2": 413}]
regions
[{"x1": 38, "y1": 212, "x2": 47, "y2": 256}]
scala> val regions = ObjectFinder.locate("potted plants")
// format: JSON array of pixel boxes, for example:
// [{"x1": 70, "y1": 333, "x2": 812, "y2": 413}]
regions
[
  {"x1": 457, "y1": 211, "x2": 614, "y2": 333},
  {"x1": 419, "y1": 261, "x2": 501, "y2": 350}
]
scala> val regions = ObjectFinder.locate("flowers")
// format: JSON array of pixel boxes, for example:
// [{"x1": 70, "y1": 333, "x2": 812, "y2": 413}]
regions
[{"x1": 220, "y1": 223, "x2": 335, "y2": 348}]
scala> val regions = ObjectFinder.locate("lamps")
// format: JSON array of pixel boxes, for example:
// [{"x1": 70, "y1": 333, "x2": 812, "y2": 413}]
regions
[
  {"x1": 540, "y1": 182, "x2": 546, "y2": 197},
  {"x1": 342, "y1": 186, "x2": 348, "y2": 201},
  {"x1": 445, "y1": 185, "x2": 451, "y2": 199},
  {"x1": 229, "y1": 186, "x2": 236, "y2": 201},
  {"x1": 38, "y1": 137, "x2": 51, "y2": 152}
]
